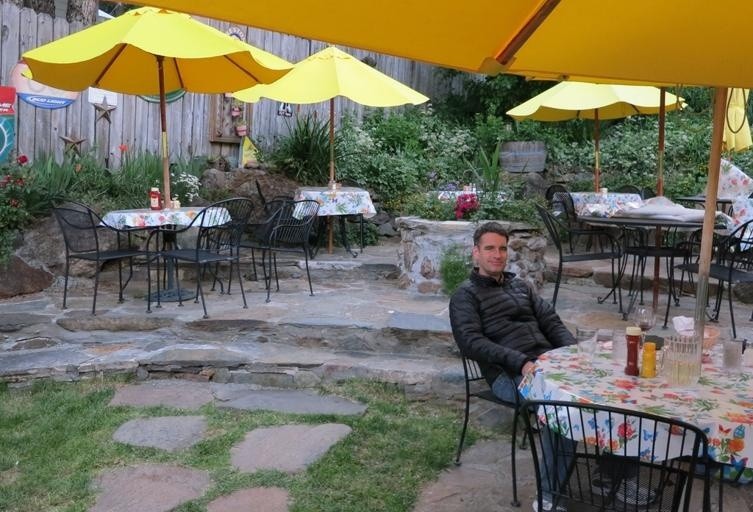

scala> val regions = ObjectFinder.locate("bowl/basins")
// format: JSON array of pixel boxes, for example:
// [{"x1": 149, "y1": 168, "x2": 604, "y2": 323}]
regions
[{"x1": 702, "y1": 325, "x2": 721, "y2": 350}]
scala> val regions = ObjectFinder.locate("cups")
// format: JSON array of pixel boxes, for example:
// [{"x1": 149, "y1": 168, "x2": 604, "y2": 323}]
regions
[
  {"x1": 723, "y1": 340, "x2": 743, "y2": 372},
  {"x1": 600, "y1": 187, "x2": 607, "y2": 200},
  {"x1": 655, "y1": 336, "x2": 700, "y2": 390},
  {"x1": 578, "y1": 327, "x2": 597, "y2": 371},
  {"x1": 613, "y1": 329, "x2": 630, "y2": 372}
]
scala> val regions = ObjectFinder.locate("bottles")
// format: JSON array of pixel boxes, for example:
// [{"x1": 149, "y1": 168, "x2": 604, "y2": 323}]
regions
[
  {"x1": 328, "y1": 180, "x2": 333, "y2": 190},
  {"x1": 640, "y1": 342, "x2": 656, "y2": 378},
  {"x1": 469, "y1": 183, "x2": 472, "y2": 192},
  {"x1": 150, "y1": 187, "x2": 162, "y2": 210},
  {"x1": 332, "y1": 181, "x2": 336, "y2": 190},
  {"x1": 473, "y1": 183, "x2": 476, "y2": 193},
  {"x1": 625, "y1": 326, "x2": 642, "y2": 376}
]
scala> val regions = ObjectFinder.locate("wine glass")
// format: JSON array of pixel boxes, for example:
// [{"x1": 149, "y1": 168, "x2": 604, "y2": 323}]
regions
[{"x1": 634, "y1": 306, "x2": 655, "y2": 349}]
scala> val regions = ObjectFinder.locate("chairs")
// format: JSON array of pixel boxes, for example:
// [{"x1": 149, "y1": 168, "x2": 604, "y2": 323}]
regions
[
  {"x1": 50, "y1": 194, "x2": 319, "y2": 319},
  {"x1": 535, "y1": 184, "x2": 753, "y2": 338},
  {"x1": 451, "y1": 332, "x2": 532, "y2": 467},
  {"x1": 521, "y1": 401, "x2": 722, "y2": 512}
]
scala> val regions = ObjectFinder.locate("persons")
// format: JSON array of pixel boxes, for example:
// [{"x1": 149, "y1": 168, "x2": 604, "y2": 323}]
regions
[{"x1": 450, "y1": 222, "x2": 656, "y2": 512}]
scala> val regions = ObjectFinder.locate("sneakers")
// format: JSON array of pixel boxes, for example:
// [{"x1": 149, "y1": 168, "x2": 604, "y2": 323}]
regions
[
  {"x1": 590, "y1": 466, "x2": 660, "y2": 507},
  {"x1": 531, "y1": 495, "x2": 568, "y2": 512}
]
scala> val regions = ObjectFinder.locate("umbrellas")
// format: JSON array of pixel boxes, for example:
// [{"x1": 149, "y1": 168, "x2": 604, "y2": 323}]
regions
[
  {"x1": 114, "y1": 1, "x2": 753, "y2": 367},
  {"x1": 721, "y1": 87, "x2": 753, "y2": 160},
  {"x1": 526, "y1": 74, "x2": 696, "y2": 198},
  {"x1": 17, "y1": 6, "x2": 295, "y2": 302},
  {"x1": 507, "y1": 76, "x2": 688, "y2": 194},
  {"x1": 228, "y1": 44, "x2": 430, "y2": 254}
]
